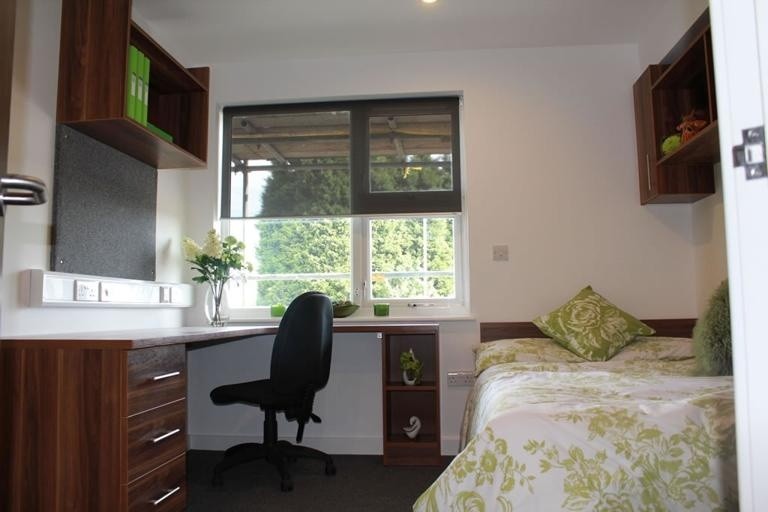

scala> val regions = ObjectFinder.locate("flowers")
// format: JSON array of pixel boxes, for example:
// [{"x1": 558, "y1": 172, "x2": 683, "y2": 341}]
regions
[{"x1": 177, "y1": 227, "x2": 254, "y2": 326}]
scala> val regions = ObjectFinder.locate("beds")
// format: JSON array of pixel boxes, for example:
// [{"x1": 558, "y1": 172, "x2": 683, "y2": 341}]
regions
[{"x1": 471, "y1": 318, "x2": 739, "y2": 512}]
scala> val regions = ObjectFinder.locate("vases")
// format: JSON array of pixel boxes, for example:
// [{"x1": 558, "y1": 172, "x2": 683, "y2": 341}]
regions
[{"x1": 203, "y1": 282, "x2": 232, "y2": 326}]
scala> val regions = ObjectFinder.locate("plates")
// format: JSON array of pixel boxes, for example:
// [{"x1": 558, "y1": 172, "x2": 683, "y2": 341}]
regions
[{"x1": 333, "y1": 304, "x2": 359, "y2": 317}]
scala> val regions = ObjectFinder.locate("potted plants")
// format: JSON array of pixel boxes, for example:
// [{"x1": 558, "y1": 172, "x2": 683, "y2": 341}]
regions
[{"x1": 399, "y1": 347, "x2": 424, "y2": 385}]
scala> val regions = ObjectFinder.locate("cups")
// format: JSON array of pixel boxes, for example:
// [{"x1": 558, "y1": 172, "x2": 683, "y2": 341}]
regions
[
  {"x1": 374, "y1": 304, "x2": 389, "y2": 315},
  {"x1": 271, "y1": 304, "x2": 286, "y2": 317}
]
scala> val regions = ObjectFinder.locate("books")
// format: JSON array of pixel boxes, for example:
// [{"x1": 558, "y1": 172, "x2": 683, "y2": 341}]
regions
[{"x1": 127, "y1": 45, "x2": 173, "y2": 143}]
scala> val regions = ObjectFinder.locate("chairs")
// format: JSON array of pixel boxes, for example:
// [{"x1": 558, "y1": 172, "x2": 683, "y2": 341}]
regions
[{"x1": 210, "y1": 291, "x2": 338, "y2": 492}]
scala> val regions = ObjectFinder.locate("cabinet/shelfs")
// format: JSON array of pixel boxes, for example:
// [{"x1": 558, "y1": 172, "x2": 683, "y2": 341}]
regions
[
  {"x1": 630, "y1": 6, "x2": 718, "y2": 205},
  {"x1": 57, "y1": 2, "x2": 211, "y2": 169}
]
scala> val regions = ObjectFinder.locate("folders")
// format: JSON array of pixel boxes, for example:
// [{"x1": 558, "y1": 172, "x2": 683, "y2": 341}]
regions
[{"x1": 126, "y1": 44, "x2": 173, "y2": 144}]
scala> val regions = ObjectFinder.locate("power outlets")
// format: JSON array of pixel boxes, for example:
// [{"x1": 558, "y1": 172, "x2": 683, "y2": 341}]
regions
[
  {"x1": 447, "y1": 371, "x2": 474, "y2": 387},
  {"x1": 75, "y1": 280, "x2": 100, "y2": 301}
]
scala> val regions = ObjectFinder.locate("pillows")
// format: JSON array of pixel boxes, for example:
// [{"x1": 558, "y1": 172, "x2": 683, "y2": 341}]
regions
[{"x1": 533, "y1": 286, "x2": 659, "y2": 362}]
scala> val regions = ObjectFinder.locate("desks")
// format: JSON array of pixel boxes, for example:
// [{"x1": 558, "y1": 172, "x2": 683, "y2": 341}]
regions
[{"x1": 0, "y1": 324, "x2": 441, "y2": 512}]
